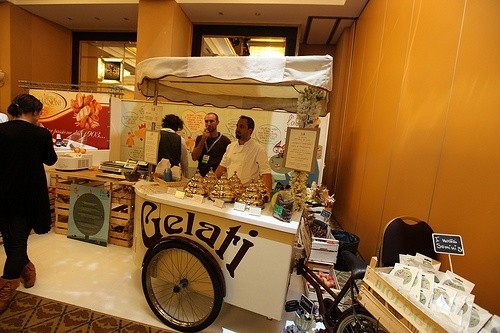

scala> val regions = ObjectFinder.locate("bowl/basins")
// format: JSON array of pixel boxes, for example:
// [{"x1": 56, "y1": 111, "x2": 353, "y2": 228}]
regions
[{"x1": 124, "y1": 172, "x2": 140, "y2": 181}]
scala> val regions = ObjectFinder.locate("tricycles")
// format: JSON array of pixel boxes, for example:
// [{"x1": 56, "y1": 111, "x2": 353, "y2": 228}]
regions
[{"x1": 133, "y1": 179, "x2": 386, "y2": 333}]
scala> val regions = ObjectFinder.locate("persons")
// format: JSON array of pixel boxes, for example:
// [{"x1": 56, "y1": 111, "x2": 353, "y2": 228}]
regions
[
  {"x1": 215, "y1": 115, "x2": 272, "y2": 204},
  {"x1": 191, "y1": 112, "x2": 232, "y2": 177},
  {"x1": 0, "y1": 94, "x2": 58, "y2": 310},
  {"x1": 151, "y1": 114, "x2": 189, "y2": 180}
]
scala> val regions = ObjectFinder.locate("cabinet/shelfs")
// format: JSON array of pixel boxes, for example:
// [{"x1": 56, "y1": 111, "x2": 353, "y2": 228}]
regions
[{"x1": 46, "y1": 168, "x2": 135, "y2": 248}]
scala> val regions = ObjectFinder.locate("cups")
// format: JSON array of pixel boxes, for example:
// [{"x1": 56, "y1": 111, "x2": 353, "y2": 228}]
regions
[{"x1": 164, "y1": 168, "x2": 172, "y2": 182}]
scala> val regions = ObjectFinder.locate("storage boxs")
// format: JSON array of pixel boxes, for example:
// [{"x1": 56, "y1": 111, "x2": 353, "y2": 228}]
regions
[
  {"x1": 57, "y1": 154, "x2": 93, "y2": 171},
  {"x1": 298, "y1": 217, "x2": 342, "y2": 302}
]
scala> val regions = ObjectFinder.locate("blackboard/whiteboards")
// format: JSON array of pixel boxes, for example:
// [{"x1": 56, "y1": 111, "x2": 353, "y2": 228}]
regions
[{"x1": 432, "y1": 233, "x2": 465, "y2": 256}]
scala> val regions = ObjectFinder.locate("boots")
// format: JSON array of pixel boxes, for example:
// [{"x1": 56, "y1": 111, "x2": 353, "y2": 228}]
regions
[
  {"x1": 19, "y1": 262, "x2": 36, "y2": 288},
  {"x1": 0, "y1": 276, "x2": 20, "y2": 313}
]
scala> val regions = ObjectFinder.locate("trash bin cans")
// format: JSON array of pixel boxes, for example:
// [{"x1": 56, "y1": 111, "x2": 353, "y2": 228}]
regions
[{"x1": 331, "y1": 230, "x2": 360, "y2": 272}]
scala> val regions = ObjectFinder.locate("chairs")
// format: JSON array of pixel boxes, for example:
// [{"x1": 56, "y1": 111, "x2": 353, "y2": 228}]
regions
[{"x1": 379, "y1": 216, "x2": 438, "y2": 268}]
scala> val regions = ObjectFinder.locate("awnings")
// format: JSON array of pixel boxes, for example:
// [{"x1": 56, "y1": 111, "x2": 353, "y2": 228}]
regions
[{"x1": 136, "y1": 56, "x2": 332, "y2": 118}]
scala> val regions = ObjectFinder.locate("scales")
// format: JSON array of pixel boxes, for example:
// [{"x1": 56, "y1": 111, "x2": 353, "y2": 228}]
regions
[{"x1": 100, "y1": 161, "x2": 125, "y2": 172}]
